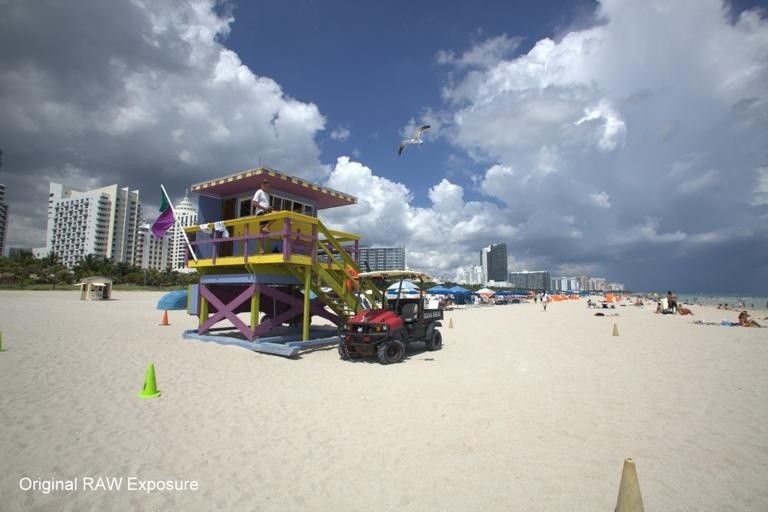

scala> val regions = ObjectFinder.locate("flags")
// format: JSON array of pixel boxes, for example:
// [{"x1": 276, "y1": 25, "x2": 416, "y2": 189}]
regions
[{"x1": 151, "y1": 188, "x2": 177, "y2": 239}]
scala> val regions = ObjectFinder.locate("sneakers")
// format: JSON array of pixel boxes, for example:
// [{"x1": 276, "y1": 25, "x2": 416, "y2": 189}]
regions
[
  {"x1": 260, "y1": 228, "x2": 270, "y2": 233},
  {"x1": 256, "y1": 248, "x2": 266, "y2": 254}
]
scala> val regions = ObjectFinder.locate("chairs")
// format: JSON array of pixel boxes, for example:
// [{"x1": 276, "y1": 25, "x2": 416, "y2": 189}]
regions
[{"x1": 382, "y1": 302, "x2": 420, "y2": 323}]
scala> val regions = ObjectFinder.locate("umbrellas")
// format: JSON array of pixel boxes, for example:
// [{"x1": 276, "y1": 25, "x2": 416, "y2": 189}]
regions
[{"x1": 387, "y1": 280, "x2": 496, "y2": 311}]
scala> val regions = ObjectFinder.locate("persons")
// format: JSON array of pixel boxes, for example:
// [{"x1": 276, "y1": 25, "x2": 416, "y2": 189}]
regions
[
  {"x1": 251, "y1": 179, "x2": 277, "y2": 254},
  {"x1": 533, "y1": 290, "x2": 764, "y2": 327}
]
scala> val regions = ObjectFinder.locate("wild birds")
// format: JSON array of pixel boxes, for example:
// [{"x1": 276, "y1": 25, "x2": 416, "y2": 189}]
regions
[{"x1": 398, "y1": 126, "x2": 430, "y2": 155}]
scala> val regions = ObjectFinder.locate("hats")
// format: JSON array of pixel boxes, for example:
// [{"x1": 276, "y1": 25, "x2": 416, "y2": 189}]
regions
[{"x1": 262, "y1": 179, "x2": 269, "y2": 183}]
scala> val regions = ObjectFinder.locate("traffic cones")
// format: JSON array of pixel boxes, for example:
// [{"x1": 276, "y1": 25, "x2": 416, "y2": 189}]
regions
[
  {"x1": 0, "y1": 331, "x2": 5, "y2": 352},
  {"x1": 615, "y1": 456, "x2": 645, "y2": 512},
  {"x1": 162, "y1": 309, "x2": 169, "y2": 325},
  {"x1": 449, "y1": 318, "x2": 454, "y2": 328},
  {"x1": 137, "y1": 361, "x2": 161, "y2": 399},
  {"x1": 612, "y1": 323, "x2": 619, "y2": 336}
]
